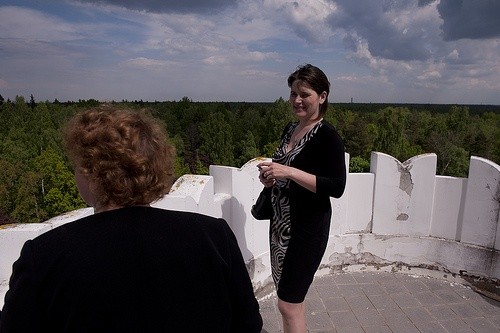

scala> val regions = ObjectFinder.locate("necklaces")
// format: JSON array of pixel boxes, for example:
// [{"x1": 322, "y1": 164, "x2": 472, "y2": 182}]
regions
[{"x1": 292, "y1": 123, "x2": 310, "y2": 148}]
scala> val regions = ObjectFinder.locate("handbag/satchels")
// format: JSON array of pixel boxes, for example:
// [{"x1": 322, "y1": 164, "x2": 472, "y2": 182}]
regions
[{"x1": 251, "y1": 185, "x2": 274, "y2": 220}]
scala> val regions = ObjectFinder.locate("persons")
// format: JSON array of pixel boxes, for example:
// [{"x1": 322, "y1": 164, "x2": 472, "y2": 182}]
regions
[
  {"x1": 256, "y1": 64, "x2": 346, "y2": 333},
  {"x1": 0, "y1": 102, "x2": 264, "y2": 333}
]
